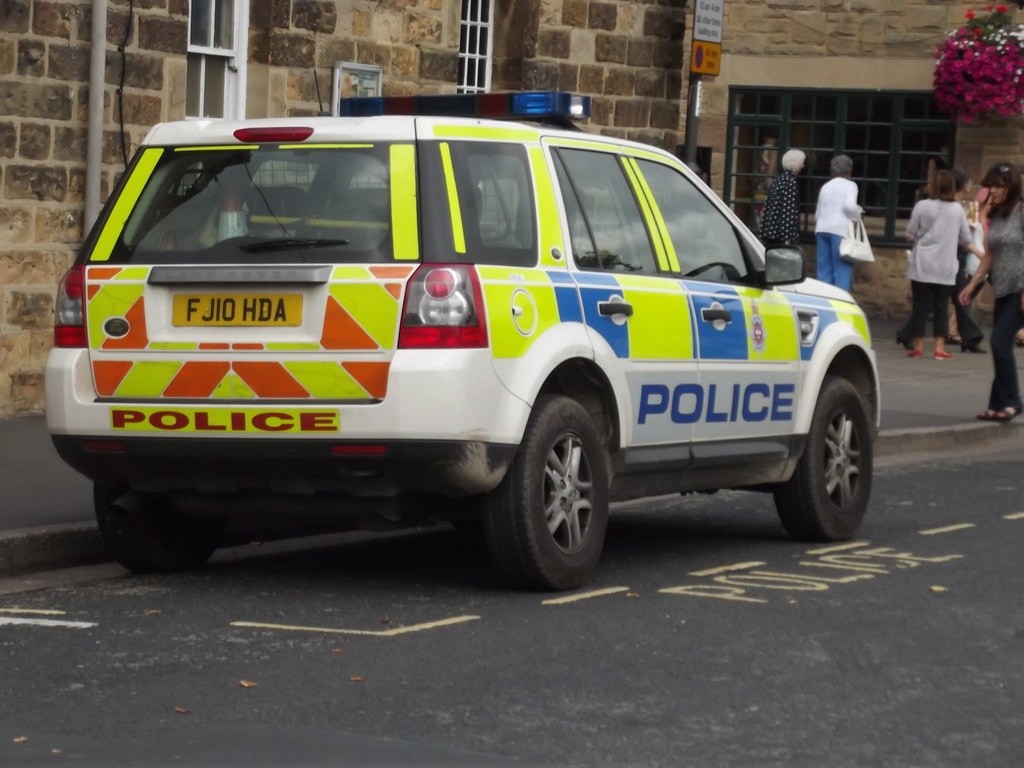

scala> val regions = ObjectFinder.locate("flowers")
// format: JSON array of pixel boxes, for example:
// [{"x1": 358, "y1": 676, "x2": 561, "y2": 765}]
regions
[{"x1": 931, "y1": 5, "x2": 1023, "y2": 123}]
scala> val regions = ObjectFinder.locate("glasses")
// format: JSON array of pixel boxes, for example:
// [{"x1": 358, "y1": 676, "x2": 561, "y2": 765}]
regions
[{"x1": 988, "y1": 164, "x2": 1011, "y2": 172}]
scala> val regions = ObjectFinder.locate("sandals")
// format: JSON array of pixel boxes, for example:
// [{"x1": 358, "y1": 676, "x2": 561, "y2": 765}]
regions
[
  {"x1": 946, "y1": 333, "x2": 962, "y2": 344},
  {"x1": 996, "y1": 405, "x2": 1023, "y2": 421},
  {"x1": 977, "y1": 409, "x2": 995, "y2": 419}
]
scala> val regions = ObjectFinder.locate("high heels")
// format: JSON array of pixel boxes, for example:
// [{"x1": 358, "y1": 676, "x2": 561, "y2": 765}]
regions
[
  {"x1": 897, "y1": 325, "x2": 913, "y2": 350},
  {"x1": 962, "y1": 344, "x2": 986, "y2": 355}
]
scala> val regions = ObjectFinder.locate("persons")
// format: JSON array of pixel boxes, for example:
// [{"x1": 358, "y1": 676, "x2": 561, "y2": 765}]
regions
[
  {"x1": 759, "y1": 147, "x2": 807, "y2": 247},
  {"x1": 928, "y1": 144, "x2": 949, "y2": 188},
  {"x1": 753, "y1": 132, "x2": 777, "y2": 230},
  {"x1": 958, "y1": 160, "x2": 1024, "y2": 421},
  {"x1": 814, "y1": 154, "x2": 866, "y2": 291},
  {"x1": 896, "y1": 164, "x2": 992, "y2": 362}
]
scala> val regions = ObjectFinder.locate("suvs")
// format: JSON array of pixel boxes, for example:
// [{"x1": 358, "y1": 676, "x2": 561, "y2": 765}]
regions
[{"x1": 44, "y1": 86, "x2": 883, "y2": 591}]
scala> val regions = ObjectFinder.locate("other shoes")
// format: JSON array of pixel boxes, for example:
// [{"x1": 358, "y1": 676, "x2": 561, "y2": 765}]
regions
[
  {"x1": 1016, "y1": 339, "x2": 1024, "y2": 346},
  {"x1": 905, "y1": 349, "x2": 924, "y2": 357},
  {"x1": 935, "y1": 352, "x2": 954, "y2": 360}
]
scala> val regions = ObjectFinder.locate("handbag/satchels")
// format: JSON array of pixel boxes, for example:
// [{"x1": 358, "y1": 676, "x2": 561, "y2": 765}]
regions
[
  {"x1": 970, "y1": 279, "x2": 994, "y2": 312},
  {"x1": 838, "y1": 217, "x2": 875, "y2": 263}
]
